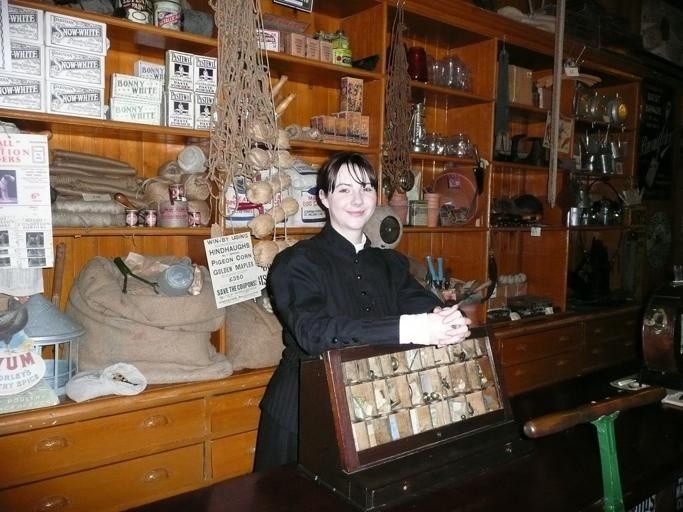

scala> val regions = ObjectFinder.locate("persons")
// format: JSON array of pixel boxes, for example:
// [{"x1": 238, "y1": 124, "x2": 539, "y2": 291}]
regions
[{"x1": 255, "y1": 151, "x2": 472, "y2": 466}]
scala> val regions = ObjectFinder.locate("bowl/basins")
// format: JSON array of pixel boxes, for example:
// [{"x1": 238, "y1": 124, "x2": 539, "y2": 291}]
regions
[{"x1": 432, "y1": 172, "x2": 478, "y2": 225}]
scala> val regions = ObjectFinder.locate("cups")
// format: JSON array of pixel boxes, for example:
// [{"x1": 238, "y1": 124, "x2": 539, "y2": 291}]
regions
[
  {"x1": 424, "y1": 193, "x2": 441, "y2": 228},
  {"x1": 426, "y1": 132, "x2": 479, "y2": 157},
  {"x1": 409, "y1": 46, "x2": 474, "y2": 92}
]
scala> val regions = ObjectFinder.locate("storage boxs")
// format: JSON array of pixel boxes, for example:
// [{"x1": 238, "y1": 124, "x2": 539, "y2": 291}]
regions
[{"x1": 507, "y1": 64, "x2": 534, "y2": 106}]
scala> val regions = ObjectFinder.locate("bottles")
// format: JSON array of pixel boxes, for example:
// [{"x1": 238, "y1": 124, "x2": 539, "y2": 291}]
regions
[
  {"x1": 126, "y1": 183, "x2": 201, "y2": 229},
  {"x1": 332, "y1": 29, "x2": 353, "y2": 68},
  {"x1": 153, "y1": 0, "x2": 182, "y2": 32}
]
